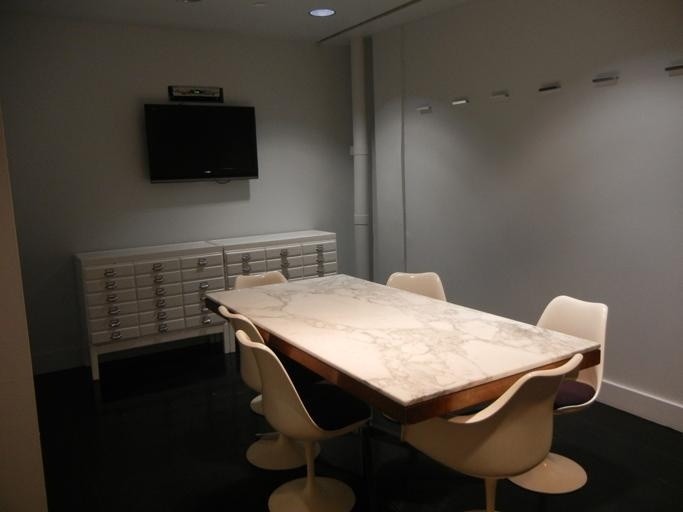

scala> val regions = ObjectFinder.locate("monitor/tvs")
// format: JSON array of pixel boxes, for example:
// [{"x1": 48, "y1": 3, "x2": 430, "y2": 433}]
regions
[{"x1": 144, "y1": 101, "x2": 259, "y2": 184}]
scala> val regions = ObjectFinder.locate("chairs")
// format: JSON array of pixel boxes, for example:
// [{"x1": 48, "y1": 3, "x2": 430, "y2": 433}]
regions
[
  {"x1": 387, "y1": 273, "x2": 447, "y2": 304},
  {"x1": 236, "y1": 274, "x2": 288, "y2": 290},
  {"x1": 505, "y1": 295, "x2": 609, "y2": 494},
  {"x1": 218, "y1": 306, "x2": 267, "y2": 415},
  {"x1": 235, "y1": 330, "x2": 373, "y2": 512},
  {"x1": 403, "y1": 353, "x2": 583, "y2": 511}
]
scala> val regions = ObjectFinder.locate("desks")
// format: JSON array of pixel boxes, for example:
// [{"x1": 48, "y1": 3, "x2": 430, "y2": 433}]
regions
[{"x1": 204, "y1": 273, "x2": 602, "y2": 428}]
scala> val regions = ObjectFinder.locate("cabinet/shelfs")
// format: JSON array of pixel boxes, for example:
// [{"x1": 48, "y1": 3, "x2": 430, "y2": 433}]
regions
[{"x1": 68, "y1": 229, "x2": 339, "y2": 382}]
